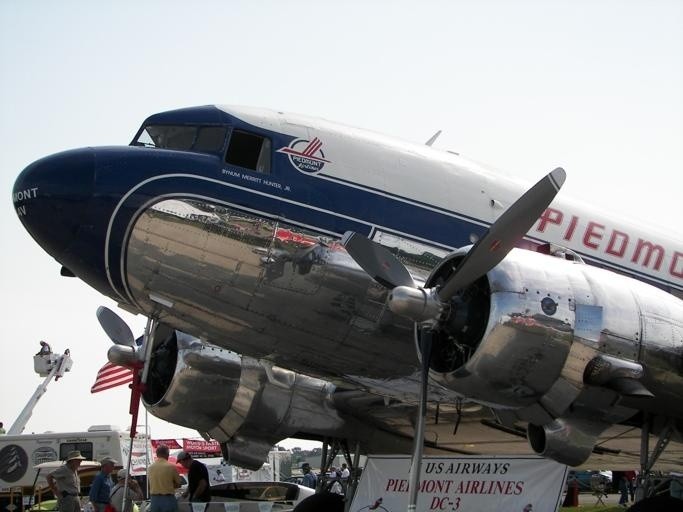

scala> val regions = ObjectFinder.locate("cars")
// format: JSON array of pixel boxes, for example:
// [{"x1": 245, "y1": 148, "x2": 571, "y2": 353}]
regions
[
  {"x1": 243, "y1": 485, "x2": 286, "y2": 500},
  {"x1": 177, "y1": 479, "x2": 315, "y2": 509},
  {"x1": 562, "y1": 467, "x2": 683, "y2": 497}
]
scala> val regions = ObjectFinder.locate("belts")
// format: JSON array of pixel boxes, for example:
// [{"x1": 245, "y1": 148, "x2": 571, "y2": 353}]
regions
[{"x1": 151, "y1": 494, "x2": 174, "y2": 497}]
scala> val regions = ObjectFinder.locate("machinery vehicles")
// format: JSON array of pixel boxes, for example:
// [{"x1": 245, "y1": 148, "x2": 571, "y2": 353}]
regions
[{"x1": 0, "y1": 349, "x2": 154, "y2": 512}]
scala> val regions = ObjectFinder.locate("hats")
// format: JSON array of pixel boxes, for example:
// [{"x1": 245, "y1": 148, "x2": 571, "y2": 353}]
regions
[
  {"x1": 66, "y1": 449, "x2": 86, "y2": 460},
  {"x1": 117, "y1": 469, "x2": 127, "y2": 481},
  {"x1": 175, "y1": 451, "x2": 189, "y2": 464},
  {"x1": 100, "y1": 456, "x2": 117, "y2": 467},
  {"x1": 299, "y1": 463, "x2": 312, "y2": 469}
]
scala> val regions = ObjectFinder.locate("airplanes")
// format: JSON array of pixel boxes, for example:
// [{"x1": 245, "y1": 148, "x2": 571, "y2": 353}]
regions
[{"x1": 10, "y1": 103, "x2": 683, "y2": 479}]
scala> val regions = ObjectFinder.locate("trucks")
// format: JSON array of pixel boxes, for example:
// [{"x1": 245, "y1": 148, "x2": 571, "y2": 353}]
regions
[{"x1": 196, "y1": 447, "x2": 367, "y2": 484}]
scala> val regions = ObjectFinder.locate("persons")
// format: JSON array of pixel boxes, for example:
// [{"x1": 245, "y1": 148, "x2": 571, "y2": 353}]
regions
[
  {"x1": 212, "y1": 469, "x2": 225, "y2": 484},
  {"x1": 47, "y1": 449, "x2": 87, "y2": 512},
  {"x1": 35, "y1": 340, "x2": 53, "y2": 357},
  {"x1": 324, "y1": 477, "x2": 343, "y2": 496},
  {"x1": 175, "y1": 451, "x2": 213, "y2": 512},
  {"x1": 329, "y1": 465, "x2": 336, "y2": 478},
  {"x1": 300, "y1": 463, "x2": 316, "y2": 488},
  {"x1": 110, "y1": 468, "x2": 145, "y2": 512},
  {"x1": 339, "y1": 463, "x2": 351, "y2": 478},
  {"x1": 89, "y1": 456, "x2": 119, "y2": 512},
  {"x1": 146, "y1": 444, "x2": 187, "y2": 512},
  {"x1": 562, "y1": 469, "x2": 637, "y2": 507},
  {"x1": 0, "y1": 422, "x2": 6, "y2": 434}
]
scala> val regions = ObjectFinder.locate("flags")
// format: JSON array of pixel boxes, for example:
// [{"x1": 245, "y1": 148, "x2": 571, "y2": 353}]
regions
[{"x1": 90, "y1": 334, "x2": 144, "y2": 394}]
